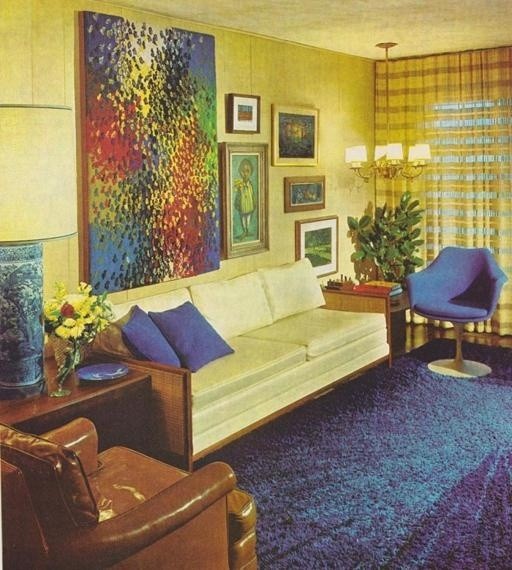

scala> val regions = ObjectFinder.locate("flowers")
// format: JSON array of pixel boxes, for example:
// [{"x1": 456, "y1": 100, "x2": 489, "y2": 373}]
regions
[{"x1": 42, "y1": 281, "x2": 114, "y2": 341}]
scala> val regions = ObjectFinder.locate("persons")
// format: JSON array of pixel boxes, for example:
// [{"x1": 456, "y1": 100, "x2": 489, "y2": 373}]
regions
[{"x1": 234, "y1": 159, "x2": 256, "y2": 241}]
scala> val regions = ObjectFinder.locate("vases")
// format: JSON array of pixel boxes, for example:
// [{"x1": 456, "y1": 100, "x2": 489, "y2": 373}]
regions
[{"x1": 50, "y1": 333, "x2": 93, "y2": 368}]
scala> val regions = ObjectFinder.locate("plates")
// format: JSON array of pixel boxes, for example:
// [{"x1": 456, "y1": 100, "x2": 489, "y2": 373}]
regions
[{"x1": 77, "y1": 365, "x2": 125, "y2": 381}]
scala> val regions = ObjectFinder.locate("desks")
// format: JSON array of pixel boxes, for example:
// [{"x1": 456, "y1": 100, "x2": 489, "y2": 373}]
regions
[
  {"x1": 1, "y1": 357, "x2": 150, "y2": 455},
  {"x1": 391, "y1": 290, "x2": 411, "y2": 348}
]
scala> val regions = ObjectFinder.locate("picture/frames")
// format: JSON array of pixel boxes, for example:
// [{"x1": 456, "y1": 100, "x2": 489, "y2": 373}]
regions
[
  {"x1": 221, "y1": 141, "x2": 270, "y2": 261},
  {"x1": 228, "y1": 93, "x2": 260, "y2": 133},
  {"x1": 272, "y1": 104, "x2": 318, "y2": 167},
  {"x1": 296, "y1": 215, "x2": 339, "y2": 279},
  {"x1": 284, "y1": 177, "x2": 325, "y2": 212}
]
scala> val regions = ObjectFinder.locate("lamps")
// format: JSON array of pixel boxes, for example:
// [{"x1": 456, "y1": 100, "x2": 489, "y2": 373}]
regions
[
  {"x1": 347, "y1": 48, "x2": 429, "y2": 181},
  {"x1": 1, "y1": 104, "x2": 76, "y2": 396}
]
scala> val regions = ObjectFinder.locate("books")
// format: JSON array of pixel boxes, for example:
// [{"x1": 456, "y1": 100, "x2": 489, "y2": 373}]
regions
[{"x1": 353, "y1": 280, "x2": 403, "y2": 297}]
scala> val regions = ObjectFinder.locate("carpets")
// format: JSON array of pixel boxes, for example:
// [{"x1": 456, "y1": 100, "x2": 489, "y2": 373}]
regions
[{"x1": 146, "y1": 337, "x2": 512, "y2": 570}]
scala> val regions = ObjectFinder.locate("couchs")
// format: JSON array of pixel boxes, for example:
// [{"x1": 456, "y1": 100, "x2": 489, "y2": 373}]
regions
[
  {"x1": 0, "y1": 417, "x2": 258, "y2": 570},
  {"x1": 93, "y1": 257, "x2": 392, "y2": 473}
]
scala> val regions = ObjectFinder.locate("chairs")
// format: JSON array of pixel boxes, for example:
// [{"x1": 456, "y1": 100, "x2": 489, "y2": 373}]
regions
[{"x1": 405, "y1": 246, "x2": 507, "y2": 376}]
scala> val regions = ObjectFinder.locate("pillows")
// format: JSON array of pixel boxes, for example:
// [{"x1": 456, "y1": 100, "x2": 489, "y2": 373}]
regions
[
  {"x1": 148, "y1": 302, "x2": 233, "y2": 371},
  {"x1": 121, "y1": 304, "x2": 181, "y2": 370}
]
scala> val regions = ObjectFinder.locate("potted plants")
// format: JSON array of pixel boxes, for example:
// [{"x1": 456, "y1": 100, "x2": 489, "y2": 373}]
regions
[{"x1": 348, "y1": 192, "x2": 424, "y2": 289}]
